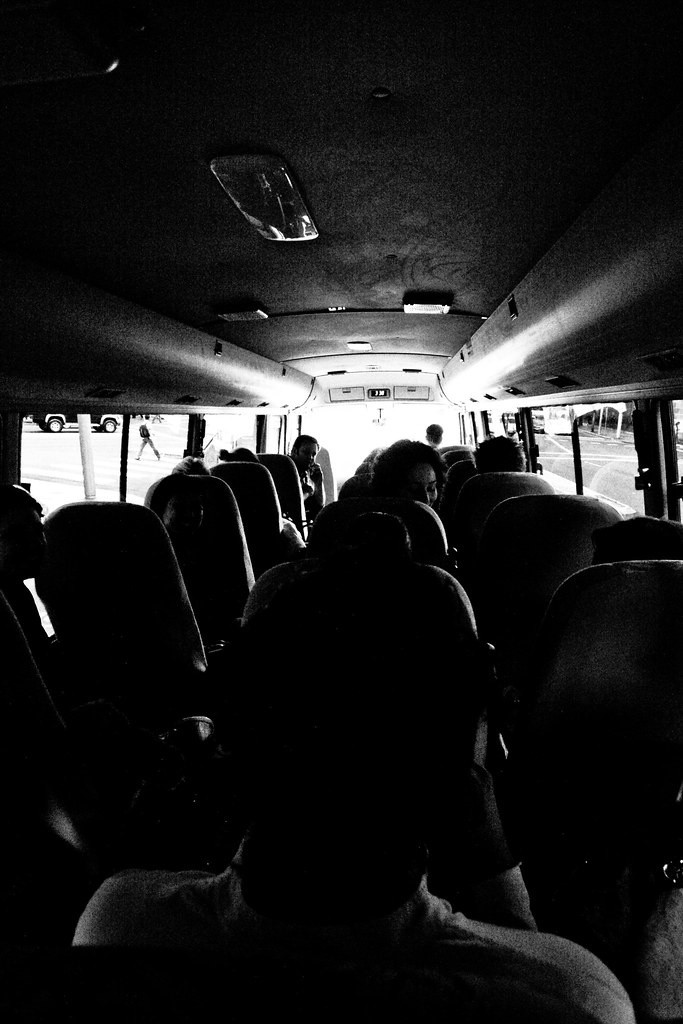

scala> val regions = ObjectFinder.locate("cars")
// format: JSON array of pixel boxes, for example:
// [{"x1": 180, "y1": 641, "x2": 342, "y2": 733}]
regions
[{"x1": 488, "y1": 411, "x2": 545, "y2": 436}]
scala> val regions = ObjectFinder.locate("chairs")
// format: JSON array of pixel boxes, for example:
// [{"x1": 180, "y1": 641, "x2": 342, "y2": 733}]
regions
[{"x1": 1, "y1": 445, "x2": 683, "y2": 1023}]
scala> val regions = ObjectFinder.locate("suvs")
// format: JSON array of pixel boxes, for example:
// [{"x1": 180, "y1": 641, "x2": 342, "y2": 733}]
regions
[{"x1": 33, "y1": 414, "x2": 123, "y2": 434}]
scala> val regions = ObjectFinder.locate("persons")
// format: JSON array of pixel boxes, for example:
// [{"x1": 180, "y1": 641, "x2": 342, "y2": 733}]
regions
[{"x1": 0, "y1": 424, "x2": 683, "y2": 1024}]
